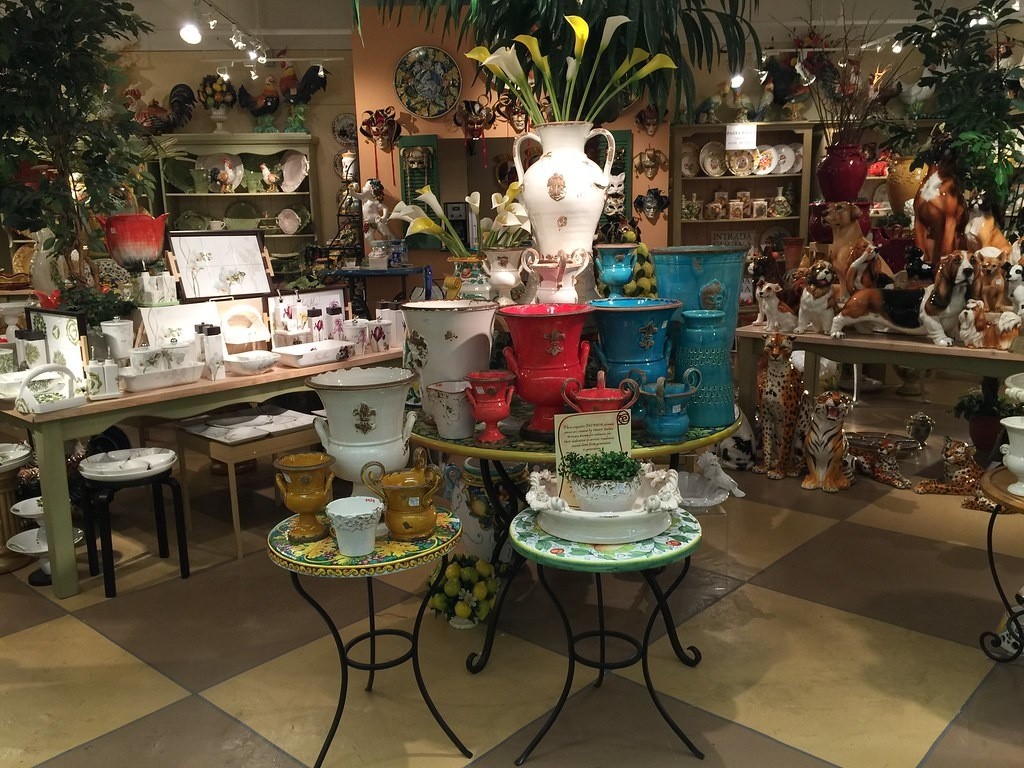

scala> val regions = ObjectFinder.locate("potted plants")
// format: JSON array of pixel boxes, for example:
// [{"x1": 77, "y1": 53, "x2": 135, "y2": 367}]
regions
[
  {"x1": 556, "y1": 444, "x2": 642, "y2": 511},
  {"x1": 946, "y1": 386, "x2": 1024, "y2": 450}
]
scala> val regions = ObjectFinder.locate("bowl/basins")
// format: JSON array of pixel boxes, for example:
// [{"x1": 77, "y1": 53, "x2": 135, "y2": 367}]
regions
[
  {"x1": 224, "y1": 349, "x2": 281, "y2": 376},
  {"x1": 118, "y1": 360, "x2": 205, "y2": 392},
  {"x1": 271, "y1": 340, "x2": 355, "y2": 368},
  {"x1": 276, "y1": 210, "x2": 300, "y2": 234}
]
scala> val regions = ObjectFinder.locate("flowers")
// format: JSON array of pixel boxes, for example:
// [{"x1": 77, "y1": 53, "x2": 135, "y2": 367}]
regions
[
  {"x1": 462, "y1": 11, "x2": 677, "y2": 122},
  {"x1": 387, "y1": 185, "x2": 534, "y2": 259}
]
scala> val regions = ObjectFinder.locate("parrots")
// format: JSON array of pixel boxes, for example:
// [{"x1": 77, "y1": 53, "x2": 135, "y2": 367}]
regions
[
  {"x1": 695, "y1": 82, "x2": 729, "y2": 124},
  {"x1": 731, "y1": 85, "x2": 757, "y2": 121},
  {"x1": 754, "y1": 76, "x2": 775, "y2": 121}
]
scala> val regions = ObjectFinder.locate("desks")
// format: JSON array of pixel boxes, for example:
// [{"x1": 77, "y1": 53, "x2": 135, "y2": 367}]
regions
[
  {"x1": 732, "y1": 323, "x2": 1024, "y2": 441},
  {"x1": 398, "y1": 397, "x2": 742, "y2": 568},
  {"x1": 265, "y1": 503, "x2": 474, "y2": 768},
  {"x1": 175, "y1": 407, "x2": 324, "y2": 561},
  {"x1": 1, "y1": 343, "x2": 403, "y2": 601},
  {"x1": 466, "y1": 507, "x2": 704, "y2": 766}
]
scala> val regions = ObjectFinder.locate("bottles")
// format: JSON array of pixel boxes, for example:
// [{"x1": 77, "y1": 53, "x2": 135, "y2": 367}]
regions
[
  {"x1": 15, "y1": 329, "x2": 47, "y2": 369},
  {"x1": 998, "y1": 416, "x2": 1024, "y2": 496},
  {"x1": 86, "y1": 356, "x2": 120, "y2": 396},
  {"x1": 194, "y1": 322, "x2": 226, "y2": 381},
  {"x1": 340, "y1": 149, "x2": 357, "y2": 181},
  {"x1": 680, "y1": 186, "x2": 798, "y2": 220}
]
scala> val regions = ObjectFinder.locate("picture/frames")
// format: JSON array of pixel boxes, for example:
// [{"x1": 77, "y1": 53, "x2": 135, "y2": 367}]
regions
[
  {"x1": 167, "y1": 230, "x2": 276, "y2": 302},
  {"x1": 21, "y1": 307, "x2": 88, "y2": 382}
]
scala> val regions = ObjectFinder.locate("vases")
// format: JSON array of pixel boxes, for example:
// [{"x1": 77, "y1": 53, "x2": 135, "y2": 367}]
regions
[
  {"x1": 94, "y1": 211, "x2": 170, "y2": 271},
  {"x1": 272, "y1": 122, "x2": 929, "y2": 558}
]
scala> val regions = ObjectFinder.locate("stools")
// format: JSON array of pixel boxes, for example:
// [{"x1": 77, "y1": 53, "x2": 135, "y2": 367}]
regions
[{"x1": 80, "y1": 449, "x2": 189, "y2": 600}]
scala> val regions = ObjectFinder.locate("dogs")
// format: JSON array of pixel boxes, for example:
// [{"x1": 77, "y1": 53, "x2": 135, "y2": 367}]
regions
[
  {"x1": 821, "y1": 201, "x2": 893, "y2": 301},
  {"x1": 958, "y1": 297, "x2": 1021, "y2": 351},
  {"x1": 831, "y1": 249, "x2": 977, "y2": 347},
  {"x1": 903, "y1": 124, "x2": 1024, "y2": 317},
  {"x1": 748, "y1": 250, "x2": 798, "y2": 332},
  {"x1": 793, "y1": 257, "x2": 842, "y2": 336}
]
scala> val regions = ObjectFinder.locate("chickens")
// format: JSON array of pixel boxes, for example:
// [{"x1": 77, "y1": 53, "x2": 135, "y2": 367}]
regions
[
  {"x1": 237, "y1": 74, "x2": 279, "y2": 118},
  {"x1": 260, "y1": 164, "x2": 284, "y2": 192},
  {"x1": 211, "y1": 157, "x2": 235, "y2": 193},
  {"x1": 892, "y1": 63, "x2": 939, "y2": 112},
  {"x1": 125, "y1": 84, "x2": 198, "y2": 136},
  {"x1": 276, "y1": 46, "x2": 331, "y2": 119},
  {"x1": 989, "y1": 42, "x2": 1024, "y2": 99}
]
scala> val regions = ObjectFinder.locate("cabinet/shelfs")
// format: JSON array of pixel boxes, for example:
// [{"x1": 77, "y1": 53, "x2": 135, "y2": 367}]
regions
[
  {"x1": 668, "y1": 121, "x2": 819, "y2": 316},
  {"x1": 73, "y1": 133, "x2": 318, "y2": 283}
]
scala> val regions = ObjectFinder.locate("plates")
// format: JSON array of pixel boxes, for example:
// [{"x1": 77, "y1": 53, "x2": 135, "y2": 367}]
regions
[
  {"x1": 331, "y1": 113, "x2": 357, "y2": 147},
  {"x1": 5, "y1": 527, "x2": 84, "y2": 556},
  {"x1": 13, "y1": 243, "x2": 35, "y2": 273},
  {"x1": 333, "y1": 147, "x2": 359, "y2": 179},
  {"x1": 760, "y1": 225, "x2": 792, "y2": 253},
  {"x1": 174, "y1": 200, "x2": 311, "y2": 235},
  {"x1": 77, "y1": 447, "x2": 179, "y2": 482},
  {"x1": 195, "y1": 153, "x2": 244, "y2": 193},
  {"x1": 10, "y1": 495, "x2": 72, "y2": 519},
  {"x1": 393, "y1": 46, "x2": 461, "y2": 119},
  {"x1": 680, "y1": 140, "x2": 804, "y2": 177},
  {"x1": 0, "y1": 442, "x2": 33, "y2": 474},
  {"x1": 278, "y1": 150, "x2": 310, "y2": 192}
]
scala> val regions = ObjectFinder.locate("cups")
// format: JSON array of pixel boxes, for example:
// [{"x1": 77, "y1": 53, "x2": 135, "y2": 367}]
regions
[
  {"x1": 341, "y1": 318, "x2": 392, "y2": 356},
  {"x1": 208, "y1": 221, "x2": 225, "y2": 232},
  {"x1": 101, "y1": 316, "x2": 190, "y2": 373}
]
